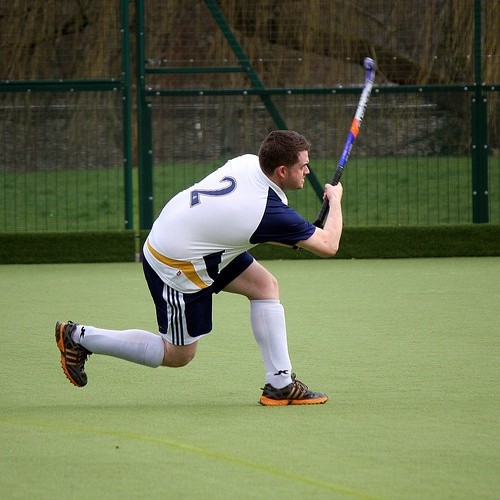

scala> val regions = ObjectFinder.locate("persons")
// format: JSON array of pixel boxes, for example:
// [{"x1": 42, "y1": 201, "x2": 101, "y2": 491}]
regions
[{"x1": 55, "y1": 130, "x2": 343, "y2": 406}]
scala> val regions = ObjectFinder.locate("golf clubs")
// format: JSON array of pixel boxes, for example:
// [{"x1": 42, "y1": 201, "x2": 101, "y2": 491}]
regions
[{"x1": 294, "y1": 56, "x2": 380, "y2": 253}]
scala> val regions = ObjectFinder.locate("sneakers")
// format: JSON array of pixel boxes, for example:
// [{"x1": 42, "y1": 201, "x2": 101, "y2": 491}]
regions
[
  {"x1": 55, "y1": 321, "x2": 89, "y2": 387},
  {"x1": 259, "y1": 373, "x2": 329, "y2": 407}
]
